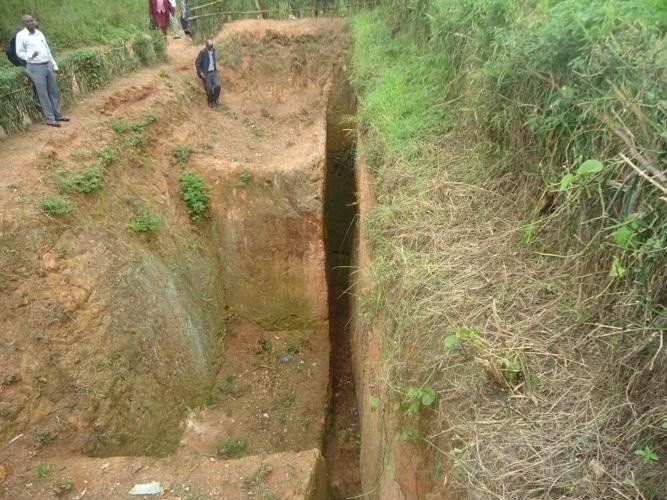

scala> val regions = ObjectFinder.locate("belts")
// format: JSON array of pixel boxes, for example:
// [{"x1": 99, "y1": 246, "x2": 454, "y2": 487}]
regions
[{"x1": 28, "y1": 61, "x2": 49, "y2": 65}]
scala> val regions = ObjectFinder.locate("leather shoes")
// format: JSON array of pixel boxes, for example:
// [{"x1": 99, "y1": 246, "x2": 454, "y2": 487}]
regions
[
  {"x1": 46, "y1": 115, "x2": 70, "y2": 127},
  {"x1": 209, "y1": 100, "x2": 219, "y2": 107}
]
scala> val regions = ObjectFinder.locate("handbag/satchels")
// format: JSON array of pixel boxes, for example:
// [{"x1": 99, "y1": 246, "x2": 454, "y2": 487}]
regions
[{"x1": 149, "y1": 19, "x2": 156, "y2": 31}]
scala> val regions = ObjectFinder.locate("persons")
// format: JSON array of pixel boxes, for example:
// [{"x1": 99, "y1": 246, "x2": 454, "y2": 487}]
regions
[
  {"x1": 5, "y1": 15, "x2": 70, "y2": 127},
  {"x1": 149, "y1": 0, "x2": 193, "y2": 40},
  {"x1": 195, "y1": 39, "x2": 221, "y2": 107}
]
